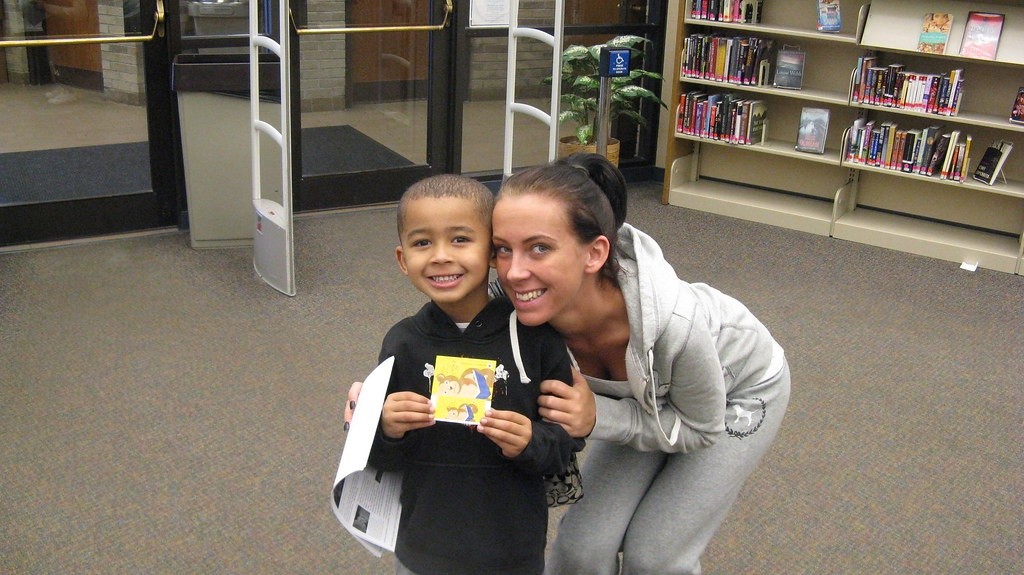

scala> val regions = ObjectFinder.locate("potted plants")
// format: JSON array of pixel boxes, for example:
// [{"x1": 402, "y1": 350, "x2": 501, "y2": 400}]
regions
[{"x1": 542, "y1": 36, "x2": 668, "y2": 170}]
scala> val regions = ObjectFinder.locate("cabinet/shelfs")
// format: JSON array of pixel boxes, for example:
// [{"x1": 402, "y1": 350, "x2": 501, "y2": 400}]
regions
[{"x1": 658, "y1": 1, "x2": 1024, "y2": 283}]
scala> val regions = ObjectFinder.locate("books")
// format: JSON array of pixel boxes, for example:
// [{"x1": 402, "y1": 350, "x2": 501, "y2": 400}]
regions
[{"x1": 677, "y1": 0, "x2": 1024, "y2": 188}]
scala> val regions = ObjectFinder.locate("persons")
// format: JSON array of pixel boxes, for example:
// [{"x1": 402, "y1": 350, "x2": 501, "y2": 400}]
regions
[
  {"x1": 342, "y1": 153, "x2": 791, "y2": 574},
  {"x1": 366, "y1": 175, "x2": 585, "y2": 575}
]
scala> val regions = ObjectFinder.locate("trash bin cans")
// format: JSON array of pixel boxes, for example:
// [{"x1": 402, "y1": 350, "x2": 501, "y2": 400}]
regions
[{"x1": 169, "y1": 50, "x2": 297, "y2": 251}]
